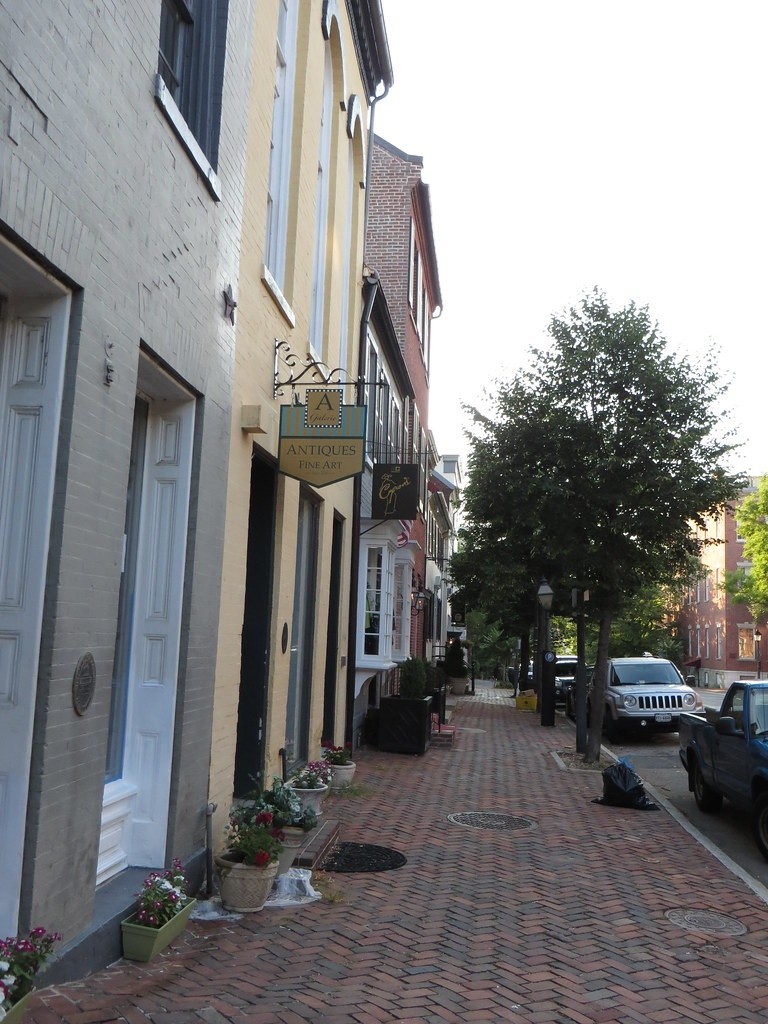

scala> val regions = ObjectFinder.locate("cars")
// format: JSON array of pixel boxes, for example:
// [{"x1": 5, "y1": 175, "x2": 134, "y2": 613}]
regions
[{"x1": 528, "y1": 654, "x2": 593, "y2": 709}]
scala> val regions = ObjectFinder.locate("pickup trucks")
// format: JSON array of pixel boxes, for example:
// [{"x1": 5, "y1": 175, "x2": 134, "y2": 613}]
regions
[{"x1": 678, "y1": 681, "x2": 768, "y2": 861}]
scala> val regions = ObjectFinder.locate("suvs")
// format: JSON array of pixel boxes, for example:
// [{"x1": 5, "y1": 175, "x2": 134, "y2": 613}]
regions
[{"x1": 587, "y1": 656, "x2": 705, "y2": 740}]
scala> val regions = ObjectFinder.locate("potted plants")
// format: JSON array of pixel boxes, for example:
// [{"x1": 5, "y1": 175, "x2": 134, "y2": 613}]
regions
[
  {"x1": 445, "y1": 639, "x2": 469, "y2": 695},
  {"x1": 381, "y1": 657, "x2": 439, "y2": 755},
  {"x1": 247, "y1": 773, "x2": 317, "y2": 875},
  {"x1": 285, "y1": 767, "x2": 326, "y2": 815}
]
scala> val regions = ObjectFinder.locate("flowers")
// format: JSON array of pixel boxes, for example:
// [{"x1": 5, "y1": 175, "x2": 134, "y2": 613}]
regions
[
  {"x1": 321, "y1": 741, "x2": 354, "y2": 765},
  {"x1": 133, "y1": 856, "x2": 187, "y2": 921},
  {"x1": 309, "y1": 762, "x2": 335, "y2": 783},
  {"x1": 0, "y1": 928, "x2": 61, "y2": 1015},
  {"x1": 224, "y1": 798, "x2": 286, "y2": 867}
]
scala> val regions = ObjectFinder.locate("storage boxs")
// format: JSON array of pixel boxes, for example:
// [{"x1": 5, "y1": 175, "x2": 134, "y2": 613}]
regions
[{"x1": 515, "y1": 697, "x2": 536, "y2": 711}]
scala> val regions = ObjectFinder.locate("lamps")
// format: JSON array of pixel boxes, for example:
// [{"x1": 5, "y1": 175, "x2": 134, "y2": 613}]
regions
[{"x1": 411, "y1": 588, "x2": 426, "y2": 616}]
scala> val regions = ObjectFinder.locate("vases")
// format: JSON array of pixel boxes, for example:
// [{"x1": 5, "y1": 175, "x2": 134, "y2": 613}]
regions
[
  {"x1": 0, "y1": 986, "x2": 36, "y2": 1024},
  {"x1": 332, "y1": 761, "x2": 357, "y2": 790},
  {"x1": 215, "y1": 853, "x2": 279, "y2": 912},
  {"x1": 326, "y1": 781, "x2": 333, "y2": 799},
  {"x1": 120, "y1": 896, "x2": 198, "y2": 962}
]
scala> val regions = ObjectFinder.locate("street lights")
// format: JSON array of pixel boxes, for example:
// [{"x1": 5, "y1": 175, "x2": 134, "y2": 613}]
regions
[
  {"x1": 753, "y1": 629, "x2": 762, "y2": 679},
  {"x1": 538, "y1": 558, "x2": 586, "y2": 754}
]
